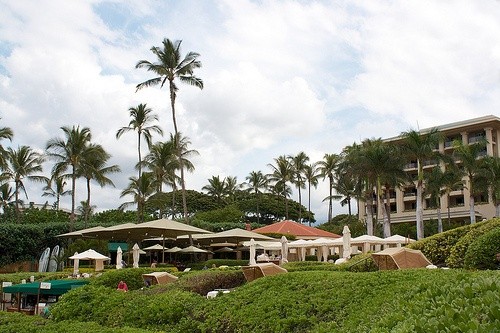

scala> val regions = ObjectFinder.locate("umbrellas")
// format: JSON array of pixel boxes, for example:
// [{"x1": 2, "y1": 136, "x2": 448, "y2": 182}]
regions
[
  {"x1": 69, "y1": 249, "x2": 110, "y2": 277},
  {"x1": 54, "y1": 215, "x2": 275, "y2": 270},
  {"x1": 343, "y1": 226, "x2": 351, "y2": 258},
  {"x1": 244, "y1": 234, "x2": 416, "y2": 262},
  {"x1": 281, "y1": 236, "x2": 288, "y2": 264}
]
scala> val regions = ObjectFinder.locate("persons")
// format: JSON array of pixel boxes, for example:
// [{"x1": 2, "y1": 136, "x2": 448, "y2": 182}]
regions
[
  {"x1": 144, "y1": 280, "x2": 151, "y2": 288},
  {"x1": 118, "y1": 280, "x2": 128, "y2": 292}
]
